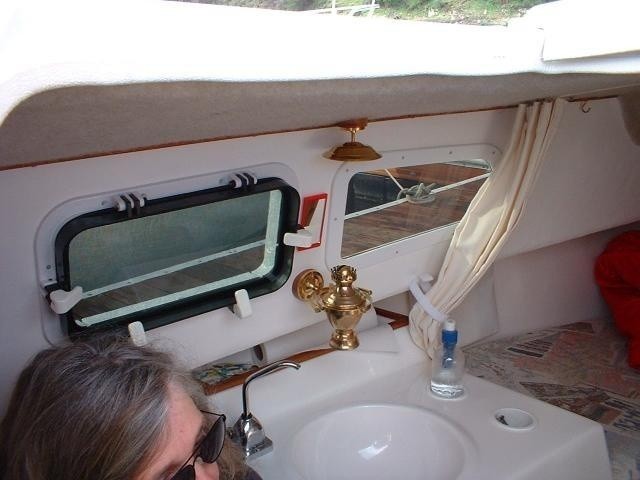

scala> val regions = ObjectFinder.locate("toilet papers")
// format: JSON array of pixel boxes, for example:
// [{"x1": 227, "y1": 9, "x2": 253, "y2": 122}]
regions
[{"x1": 251, "y1": 303, "x2": 404, "y2": 369}]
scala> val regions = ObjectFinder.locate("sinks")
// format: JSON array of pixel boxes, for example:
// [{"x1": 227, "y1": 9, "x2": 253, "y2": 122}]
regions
[{"x1": 290, "y1": 402, "x2": 477, "y2": 480}]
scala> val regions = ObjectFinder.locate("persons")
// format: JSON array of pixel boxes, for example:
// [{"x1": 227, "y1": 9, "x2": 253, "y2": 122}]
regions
[{"x1": 0, "y1": 329, "x2": 263, "y2": 480}]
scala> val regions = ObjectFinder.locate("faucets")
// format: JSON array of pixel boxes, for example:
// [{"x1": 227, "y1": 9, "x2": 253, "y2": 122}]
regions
[{"x1": 228, "y1": 359, "x2": 301, "y2": 462}]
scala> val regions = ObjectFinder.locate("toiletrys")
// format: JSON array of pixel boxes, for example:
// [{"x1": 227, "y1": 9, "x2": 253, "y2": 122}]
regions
[{"x1": 430, "y1": 318, "x2": 467, "y2": 398}]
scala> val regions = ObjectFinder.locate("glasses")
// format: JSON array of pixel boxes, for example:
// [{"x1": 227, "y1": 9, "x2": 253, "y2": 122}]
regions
[{"x1": 170, "y1": 410, "x2": 226, "y2": 480}]
